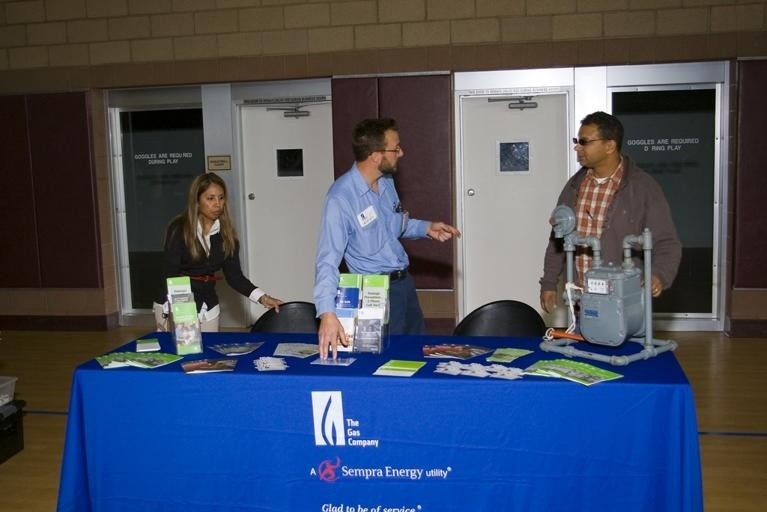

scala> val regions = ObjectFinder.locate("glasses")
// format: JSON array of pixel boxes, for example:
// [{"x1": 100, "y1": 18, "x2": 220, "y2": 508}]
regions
[
  {"x1": 573, "y1": 137, "x2": 606, "y2": 145},
  {"x1": 373, "y1": 146, "x2": 401, "y2": 154}
]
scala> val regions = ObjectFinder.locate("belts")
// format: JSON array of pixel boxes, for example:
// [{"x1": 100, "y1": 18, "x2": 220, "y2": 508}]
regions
[
  {"x1": 385, "y1": 271, "x2": 407, "y2": 281},
  {"x1": 187, "y1": 274, "x2": 224, "y2": 282}
]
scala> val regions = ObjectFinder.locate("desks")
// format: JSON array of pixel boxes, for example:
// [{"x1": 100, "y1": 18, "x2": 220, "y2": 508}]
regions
[{"x1": 78, "y1": 331, "x2": 690, "y2": 512}]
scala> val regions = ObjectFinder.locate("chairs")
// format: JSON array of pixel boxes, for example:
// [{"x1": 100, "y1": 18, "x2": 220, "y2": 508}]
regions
[
  {"x1": 453, "y1": 300, "x2": 548, "y2": 338},
  {"x1": 252, "y1": 302, "x2": 321, "y2": 335}
]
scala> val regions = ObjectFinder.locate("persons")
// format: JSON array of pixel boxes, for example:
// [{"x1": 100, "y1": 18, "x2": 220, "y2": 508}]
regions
[
  {"x1": 312, "y1": 118, "x2": 460, "y2": 361},
  {"x1": 540, "y1": 112, "x2": 682, "y2": 335},
  {"x1": 153, "y1": 173, "x2": 283, "y2": 332}
]
